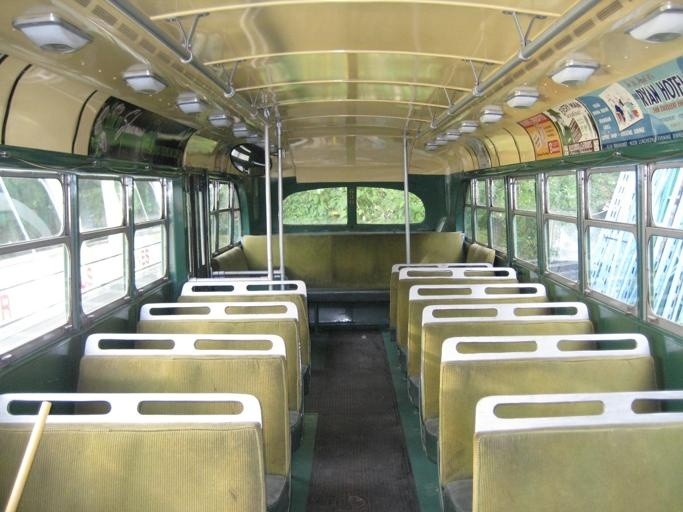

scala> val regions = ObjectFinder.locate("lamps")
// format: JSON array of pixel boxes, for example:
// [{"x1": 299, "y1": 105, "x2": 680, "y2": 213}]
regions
[
  {"x1": 11, "y1": 6, "x2": 90, "y2": 56},
  {"x1": 424, "y1": 52, "x2": 601, "y2": 152},
  {"x1": 613, "y1": 1, "x2": 683, "y2": 44},
  {"x1": 120, "y1": 61, "x2": 276, "y2": 156}
]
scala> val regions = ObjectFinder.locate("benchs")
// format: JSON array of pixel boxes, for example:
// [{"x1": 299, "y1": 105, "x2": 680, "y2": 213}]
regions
[
  {"x1": 137, "y1": 246, "x2": 309, "y2": 450},
  {"x1": 77, "y1": 334, "x2": 292, "y2": 512},
  {"x1": 1, "y1": 392, "x2": 268, "y2": 510},
  {"x1": 390, "y1": 265, "x2": 596, "y2": 463},
  {"x1": 434, "y1": 331, "x2": 661, "y2": 511},
  {"x1": 240, "y1": 230, "x2": 467, "y2": 298},
  {"x1": 471, "y1": 389, "x2": 682, "y2": 512}
]
